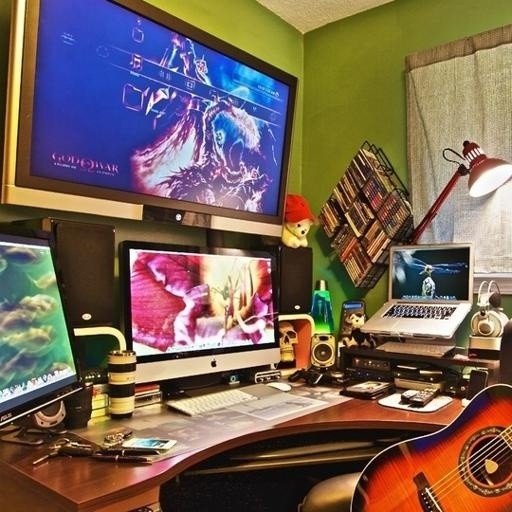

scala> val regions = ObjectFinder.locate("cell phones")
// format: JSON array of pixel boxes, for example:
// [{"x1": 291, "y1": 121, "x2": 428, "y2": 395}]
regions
[
  {"x1": 122, "y1": 437, "x2": 176, "y2": 450},
  {"x1": 466, "y1": 370, "x2": 488, "y2": 400}
]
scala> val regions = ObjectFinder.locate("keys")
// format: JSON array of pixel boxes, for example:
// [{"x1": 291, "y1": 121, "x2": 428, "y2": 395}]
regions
[{"x1": 31, "y1": 429, "x2": 133, "y2": 466}]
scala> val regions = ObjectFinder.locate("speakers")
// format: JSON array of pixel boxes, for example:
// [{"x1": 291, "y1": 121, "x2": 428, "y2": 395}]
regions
[
  {"x1": 31, "y1": 395, "x2": 66, "y2": 429},
  {"x1": 310, "y1": 333, "x2": 335, "y2": 369},
  {"x1": 276, "y1": 246, "x2": 312, "y2": 314},
  {"x1": 12, "y1": 218, "x2": 115, "y2": 327}
]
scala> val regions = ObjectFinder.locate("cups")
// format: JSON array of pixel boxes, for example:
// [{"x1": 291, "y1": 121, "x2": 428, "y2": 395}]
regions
[
  {"x1": 63, "y1": 381, "x2": 94, "y2": 430},
  {"x1": 106, "y1": 349, "x2": 136, "y2": 418}
]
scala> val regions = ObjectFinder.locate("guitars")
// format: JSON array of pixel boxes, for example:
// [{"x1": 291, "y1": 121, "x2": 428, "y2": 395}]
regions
[{"x1": 350, "y1": 384, "x2": 512, "y2": 511}]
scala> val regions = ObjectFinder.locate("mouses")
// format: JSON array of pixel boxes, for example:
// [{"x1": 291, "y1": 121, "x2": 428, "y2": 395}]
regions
[{"x1": 267, "y1": 382, "x2": 291, "y2": 392}]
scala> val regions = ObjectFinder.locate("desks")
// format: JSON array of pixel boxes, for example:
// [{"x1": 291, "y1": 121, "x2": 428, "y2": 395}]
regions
[{"x1": 0, "y1": 373, "x2": 464, "y2": 512}]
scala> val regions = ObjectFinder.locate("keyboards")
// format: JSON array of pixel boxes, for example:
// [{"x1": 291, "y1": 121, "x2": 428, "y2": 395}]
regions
[
  {"x1": 229, "y1": 429, "x2": 374, "y2": 461},
  {"x1": 165, "y1": 388, "x2": 258, "y2": 416}
]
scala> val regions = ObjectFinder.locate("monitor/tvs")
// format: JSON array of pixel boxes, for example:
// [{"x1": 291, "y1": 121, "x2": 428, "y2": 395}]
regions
[
  {"x1": 0, "y1": 0, "x2": 297, "y2": 238},
  {"x1": 0, "y1": 234, "x2": 85, "y2": 425},
  {"x1": 119, "y1": 240, "x2": 281, "y2": 385}
]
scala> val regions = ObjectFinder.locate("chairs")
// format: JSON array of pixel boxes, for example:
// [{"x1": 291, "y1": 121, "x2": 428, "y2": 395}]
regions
[{"x1": 298, "y1": 472, "x2": 361, "y2": 512}]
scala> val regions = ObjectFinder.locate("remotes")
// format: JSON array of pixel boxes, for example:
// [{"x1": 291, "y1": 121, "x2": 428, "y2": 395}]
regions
[{"x1": 409, "y1": 387, "x2": 439, "y2": 406}]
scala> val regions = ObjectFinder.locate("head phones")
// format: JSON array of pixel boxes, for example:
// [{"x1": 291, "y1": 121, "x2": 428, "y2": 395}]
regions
[{"x1": 470, "y1": 280, "x2": 510, "y2": 336}]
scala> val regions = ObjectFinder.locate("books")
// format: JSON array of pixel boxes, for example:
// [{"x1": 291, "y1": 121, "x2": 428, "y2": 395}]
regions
[
  {"x1": 318, "y1": 149, "x2": 414, "y2": 291},
  {"x1": 64, "y1": 383, "x2": 162, "y2": 425}
]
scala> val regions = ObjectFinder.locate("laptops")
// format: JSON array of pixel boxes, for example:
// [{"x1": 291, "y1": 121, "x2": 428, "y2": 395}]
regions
[{"x1": 360, "y1": 243, "x2": 474, "y2": 339}]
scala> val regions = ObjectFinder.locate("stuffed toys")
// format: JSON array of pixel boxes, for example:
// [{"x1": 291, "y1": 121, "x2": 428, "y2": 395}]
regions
[
  {"x1": 342, "y1": 311, "x2": 377, "y2": 349},
  {"x1": 281, "y1": 193, "x2": 319, "y2": 249}
]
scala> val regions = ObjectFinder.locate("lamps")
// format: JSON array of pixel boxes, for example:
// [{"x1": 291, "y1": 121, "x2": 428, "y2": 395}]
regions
[{"x1": 407, "y1": 141, "x2": 512, "y2": 244}]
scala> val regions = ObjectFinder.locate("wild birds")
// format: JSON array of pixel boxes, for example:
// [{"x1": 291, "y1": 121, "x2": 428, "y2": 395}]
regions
[{"x1": 419, "y1": 265, "x2": 435, "y2": 296}]
